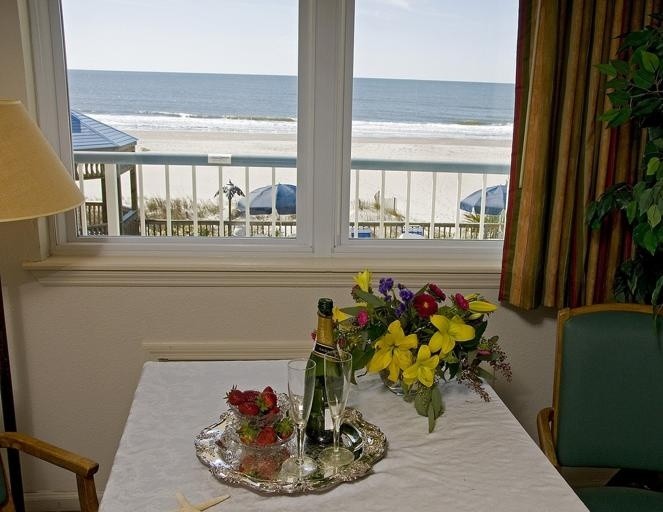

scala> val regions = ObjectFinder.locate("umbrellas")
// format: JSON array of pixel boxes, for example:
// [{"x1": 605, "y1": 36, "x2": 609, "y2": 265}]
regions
[
  {"x1": 239, "y1": 184, "x2": 296, "y2": 231},
  {"x1": 459, "y1": 182, "x2": 507, "y2": 216}
]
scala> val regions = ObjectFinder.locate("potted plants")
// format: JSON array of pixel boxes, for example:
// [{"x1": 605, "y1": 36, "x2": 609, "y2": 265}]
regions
[{"x1": 576, "y1": 13, "x2": 663, "y2": 493}]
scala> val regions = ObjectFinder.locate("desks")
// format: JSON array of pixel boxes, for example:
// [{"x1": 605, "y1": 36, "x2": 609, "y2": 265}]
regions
[{"x1": 97, "y1": 361, "x2": 591, "y2": 512}]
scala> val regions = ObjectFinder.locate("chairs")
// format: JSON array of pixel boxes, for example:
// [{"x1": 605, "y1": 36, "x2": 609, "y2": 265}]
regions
[
  {"x1": 0, "y1": 432, "x2": 100, "y2": 512},
  {"x1": 538, "y1": 301, "x2": 663, "y2": 512}
]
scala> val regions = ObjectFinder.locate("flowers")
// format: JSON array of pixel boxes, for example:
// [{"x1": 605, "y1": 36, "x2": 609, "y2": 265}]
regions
[{"x1": 309, "y1": 267, "x2": 513, "y2": 434}]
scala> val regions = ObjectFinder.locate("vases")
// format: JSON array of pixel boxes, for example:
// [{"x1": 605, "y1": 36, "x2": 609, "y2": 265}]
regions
[{"x1": 379, "y1": 368, "x2": 440, "y2": 396}]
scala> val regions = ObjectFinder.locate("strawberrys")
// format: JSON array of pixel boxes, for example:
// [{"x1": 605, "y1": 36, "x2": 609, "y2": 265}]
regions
[{"x1": 224, "y1": 386, "x2": 294, "y2": 479}]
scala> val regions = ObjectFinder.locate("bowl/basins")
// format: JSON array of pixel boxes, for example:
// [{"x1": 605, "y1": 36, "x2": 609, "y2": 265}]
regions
[{"x1": 227, "y1": 395, "x2": 296, "y2": 449}]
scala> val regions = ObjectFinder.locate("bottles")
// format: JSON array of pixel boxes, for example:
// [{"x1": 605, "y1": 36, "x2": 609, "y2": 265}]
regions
[{"x1": 304, "y1": 298, "x2": 345, "y2": 447}]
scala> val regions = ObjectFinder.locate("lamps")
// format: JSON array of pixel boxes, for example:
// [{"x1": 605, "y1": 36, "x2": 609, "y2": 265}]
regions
[{"x1": 0, "y1": 98, "x2": 87, "y2": 512}]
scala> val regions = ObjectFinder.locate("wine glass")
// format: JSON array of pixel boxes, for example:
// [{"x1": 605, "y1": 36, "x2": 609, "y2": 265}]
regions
[{"x1": 278, "y1": 351, "x2": 355, "y2": 479}]
